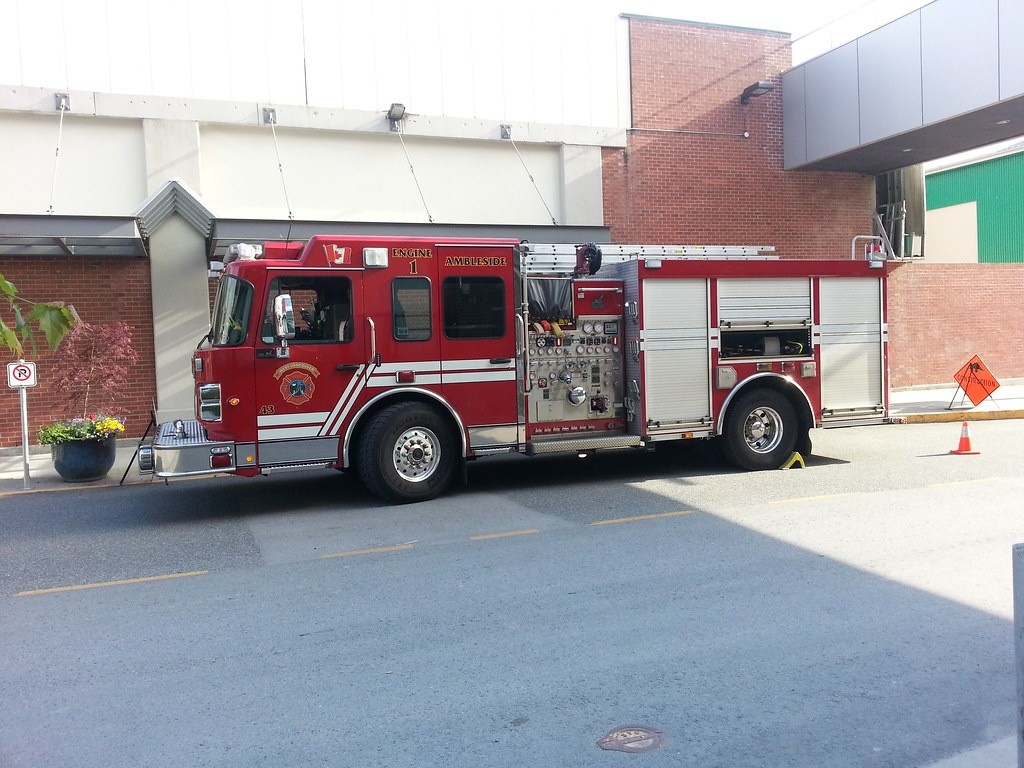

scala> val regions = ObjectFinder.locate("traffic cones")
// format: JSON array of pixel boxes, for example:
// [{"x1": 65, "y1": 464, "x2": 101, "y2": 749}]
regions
[{"x1": 950, "y1": 421, "x2": 980, "y2": 455}]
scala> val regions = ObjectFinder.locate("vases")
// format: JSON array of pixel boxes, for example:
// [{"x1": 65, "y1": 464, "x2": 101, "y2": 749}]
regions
[{"x1": 51, "y1": 437, "x2": 116, "y2": 482}]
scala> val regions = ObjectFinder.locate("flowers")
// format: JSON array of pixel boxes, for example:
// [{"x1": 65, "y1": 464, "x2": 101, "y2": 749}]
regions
[{"x1": 36, "y1": 411, "x2": 128, "y2": 447}]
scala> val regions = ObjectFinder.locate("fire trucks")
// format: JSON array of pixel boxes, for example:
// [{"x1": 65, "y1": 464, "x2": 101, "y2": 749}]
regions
[{"x1": 135, "y1": 231, "x2": 908, "y2": 505}]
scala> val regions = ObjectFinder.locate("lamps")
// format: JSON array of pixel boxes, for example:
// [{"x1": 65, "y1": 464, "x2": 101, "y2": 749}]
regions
[
  {"x1": 386, "y1": 103, "x2": 405, "y2": 123},
  {"x1": 740, "y1": 82, "x2": 775, "y2": 104}
]
